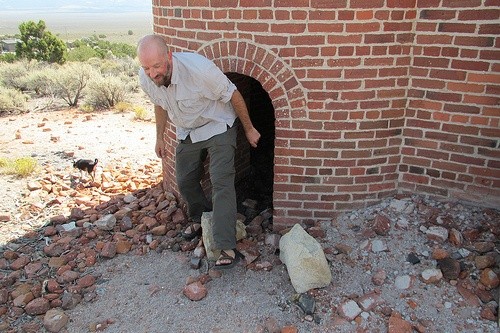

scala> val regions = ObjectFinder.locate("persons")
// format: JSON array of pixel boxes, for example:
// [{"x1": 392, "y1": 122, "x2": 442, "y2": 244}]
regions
[{"x1": 135, "y1": 34, "x2": 262, "y2": 269}]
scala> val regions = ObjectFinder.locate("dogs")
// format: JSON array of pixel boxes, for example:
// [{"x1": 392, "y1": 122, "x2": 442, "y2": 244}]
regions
[{"x1": 73, "y1": 158, "x2": 98, "y2": 183}]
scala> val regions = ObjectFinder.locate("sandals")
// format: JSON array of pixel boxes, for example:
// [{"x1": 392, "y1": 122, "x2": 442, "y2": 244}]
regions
[
  {"x1": 215, "y1": 249, "x2": 239, "y2": 269},
  {"x1": 182, "y1": 221, "x2": 202, "y2": 237}
]
